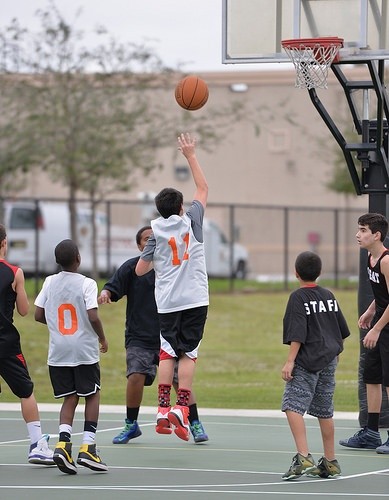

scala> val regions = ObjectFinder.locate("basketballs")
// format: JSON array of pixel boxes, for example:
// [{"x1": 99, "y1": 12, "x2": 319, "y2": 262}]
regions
[{"x1": 174, "y1": 75, "x2": 210, "y2": 111}]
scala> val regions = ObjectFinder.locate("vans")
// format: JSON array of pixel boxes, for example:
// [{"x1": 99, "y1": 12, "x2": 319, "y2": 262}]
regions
[{"x1": 0, "y1": 200, "x2": 116, "y2": 277}]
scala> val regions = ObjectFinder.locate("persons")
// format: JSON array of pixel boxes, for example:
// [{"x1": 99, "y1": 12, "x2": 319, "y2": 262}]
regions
[
  {"x1": 0, "y1": 224, "x2": 57, "y2": 467},
  {"x1": 281, "y1": 250, "x2": 350, "y2": 482},
  {"x1": 97, "y1": 227, "x2": 208, "y2": 445},
  {"x1": 338, "y1": 214, "x2": 389, "y2": 455},
  {"x1": 34, "y1": 239, "x2": 107, "y2": 475},
  {"x1": 136, "y1": 133, "x2": 209, "y2": 441}
]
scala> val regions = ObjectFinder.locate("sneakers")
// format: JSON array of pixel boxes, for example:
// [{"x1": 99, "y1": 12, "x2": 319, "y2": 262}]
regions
[
  {"x1": 376, "y1": 430, "x2": 389, "y2": 454},
  {"x1": 305, "y1": 456, "x2": 342, "y2": 477},
  {"x1": 113, "y1": 418, "x2": 142, "y2": 444},
  {"x1": 155, "y1": 405, "x2": 173, "y2": 435},
  {"x1": 339, "y1": 427, "x2": 382, "y2": 450},
  {"x1": 28, "y1": 434, "x2": 57, "y2": 466},
  {"x1": 189, "y1": 421, "x2": 208, "y2": 443},
  {"x1": 168, "y1": 405, "x2": 191, "y2": 441},
  {"x1": 280, "y1": 453, "x2": 317, "y2": 480},
  {"x1": 77, "y1": 444, "x2": 108, "y2": 471},
  {"x1": 53, "y1": 440, "x2": 77, "y2": 475}
]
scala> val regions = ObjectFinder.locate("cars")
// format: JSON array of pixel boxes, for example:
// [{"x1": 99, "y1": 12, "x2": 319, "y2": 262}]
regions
[{"x1": 136, "y1": 212, "x2": 247, "y2": 280}]
